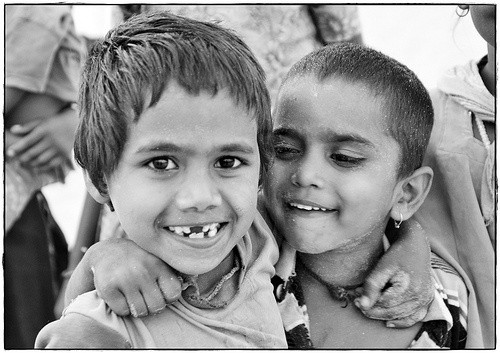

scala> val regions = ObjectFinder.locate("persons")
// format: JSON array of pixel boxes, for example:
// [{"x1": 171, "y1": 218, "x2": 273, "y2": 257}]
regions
[{"x1": 5, "y1": 4, "x2": 496, "y2": 350}]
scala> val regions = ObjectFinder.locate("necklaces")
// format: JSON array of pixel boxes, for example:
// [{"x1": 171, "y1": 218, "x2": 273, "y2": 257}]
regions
[{"x1": 297, "y1": 260, "x2": 367, "y2": 309}]
